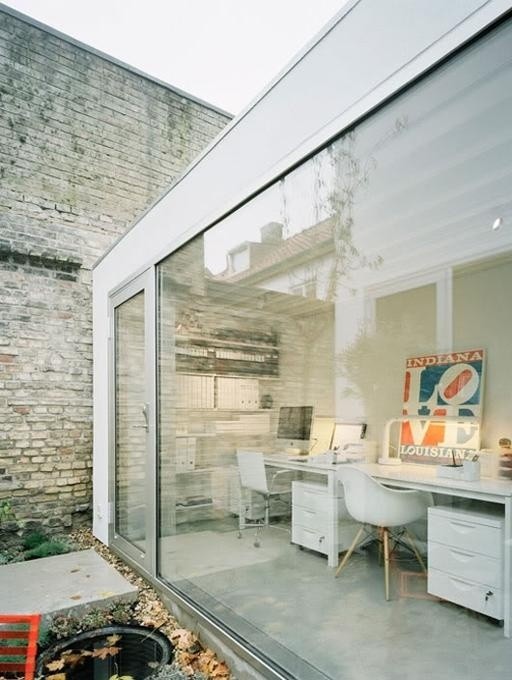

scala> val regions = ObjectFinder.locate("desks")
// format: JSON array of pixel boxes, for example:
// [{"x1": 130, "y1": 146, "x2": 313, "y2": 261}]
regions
[
  {"x1": 330, "y1": 459, "x2": 512, "y2": 639},
  {"x1": 228, "y1": 445, "x2": 338, "y2": 568}
]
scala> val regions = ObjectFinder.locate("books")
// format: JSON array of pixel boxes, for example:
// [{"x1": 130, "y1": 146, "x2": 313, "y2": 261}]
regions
[{"x1": 176, "y1": 343, "x2": 279, "y2": 510}]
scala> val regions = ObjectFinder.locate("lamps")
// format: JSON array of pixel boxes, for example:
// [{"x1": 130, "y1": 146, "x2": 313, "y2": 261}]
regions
[{"x1": 374, "y1": 414, "x2": 483, "y2": 466}]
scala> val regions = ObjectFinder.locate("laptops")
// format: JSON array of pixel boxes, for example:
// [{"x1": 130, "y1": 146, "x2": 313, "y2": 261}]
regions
[{"x1": 308, "y1": 422, "x2": 368, "y2": 464}]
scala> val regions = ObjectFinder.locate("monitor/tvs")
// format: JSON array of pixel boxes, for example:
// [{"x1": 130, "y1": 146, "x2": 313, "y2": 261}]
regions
[{"x1": 274, "y1": 406, "x2": 315, "y2": 456}]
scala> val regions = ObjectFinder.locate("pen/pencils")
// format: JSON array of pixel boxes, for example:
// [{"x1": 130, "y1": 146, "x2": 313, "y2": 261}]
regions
[{"x1": 452, "y1": 451, "x2": 456, "y2": 468}]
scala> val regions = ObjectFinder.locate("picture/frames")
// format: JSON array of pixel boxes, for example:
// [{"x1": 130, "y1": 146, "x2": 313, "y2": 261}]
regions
[{"x1": 329, "y1": 422, "x2": 367, "y2": 451}]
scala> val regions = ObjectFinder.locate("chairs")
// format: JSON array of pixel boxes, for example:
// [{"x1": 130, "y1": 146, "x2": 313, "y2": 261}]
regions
[
  {"x1": 235, "y1": 447, "x2": 300, "y2": 549},
  {"x1": 330, "y1": 464, "x2": 437, "y2": 603},
  {"x1": 0, "y1": 612, "x2": 43, "y2": 680}
]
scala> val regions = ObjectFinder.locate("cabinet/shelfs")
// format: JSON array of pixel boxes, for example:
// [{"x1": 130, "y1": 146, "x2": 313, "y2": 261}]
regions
[
  {"x1": 290, "y1": 480, "x2": 375, "y2": 560},
  {"x1": 174, "y1": 324, "x2": 282, "y2": 384},
  {"x1": 174, "y1": 429, "x2": 223, "y2": 514},
  {"x1": 428, "y1": 497, "x2": 506, "y2": 627}
]
